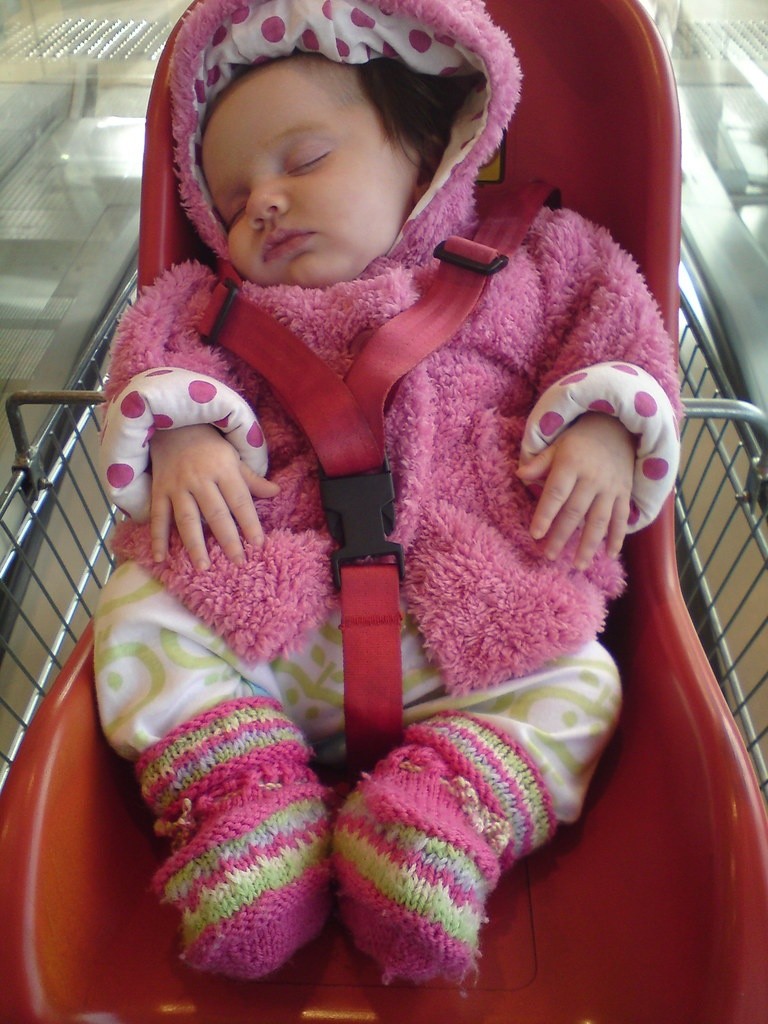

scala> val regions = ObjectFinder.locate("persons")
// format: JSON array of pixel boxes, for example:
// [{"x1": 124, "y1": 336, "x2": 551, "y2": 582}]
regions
[{"x1": 93, "y1": 0, "x2": 688, "y2": 985}]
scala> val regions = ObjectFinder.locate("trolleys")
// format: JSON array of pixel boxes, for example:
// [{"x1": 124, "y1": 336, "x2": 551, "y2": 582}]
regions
[{"x1": 0, "y1": 284, "x2": 767, "y2": 1024}]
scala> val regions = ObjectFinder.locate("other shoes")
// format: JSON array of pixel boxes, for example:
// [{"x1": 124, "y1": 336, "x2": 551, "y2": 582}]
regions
[
  {"x1": 136, "y1": 696, "x2": 335, "y2": 979},
  {"x1": 330, "y1": 709, "x2": 557, "y2": 986}
]
